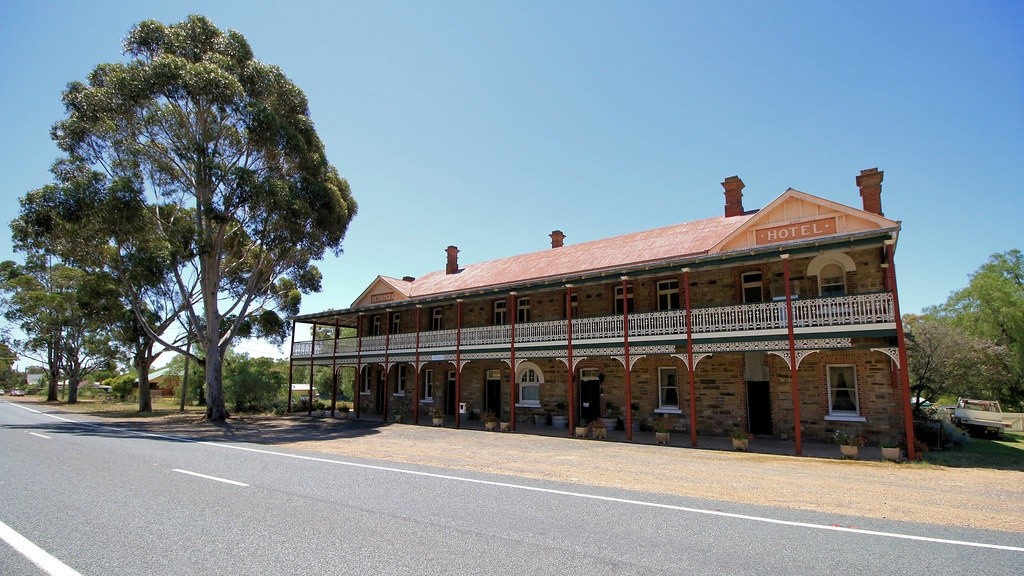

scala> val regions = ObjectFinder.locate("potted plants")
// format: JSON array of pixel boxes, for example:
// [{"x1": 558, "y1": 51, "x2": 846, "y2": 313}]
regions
[
  {"x1": 338, "y1": 406, "x2": 350, "y2": 417},
  {"x1": 879, "y1": 428, "x2": 900, "y2": 462},
  {"x1": 500, "y1": 411, "x2": 512, "y2": 431},
  {"x1": 598, "y1": 402, "x2": 618, "y2": 432},
  {"x1": 621, "y1": 403, "x2": 642, "y2": 432},
  {"x1": 575, "y1": 418, "x2": 589, "y2": 437},
  {"x1": 314, "y1": 402, "x2": 325, "y2": 416},
  {"x1": 551, "y1": 403, "x2": 568, "y2": 429},
  {"x1": 392, "y1": 406, "x2": 404, "y2": 423}
]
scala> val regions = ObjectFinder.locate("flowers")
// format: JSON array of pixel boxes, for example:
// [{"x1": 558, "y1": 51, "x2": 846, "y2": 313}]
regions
[
  {"x1": 480, "y1": 408, "x2": 498, "y2": 423},
  {"x1": 652, "y1": 418, "x2": 673, "y2": 433},
  {"x1": 832, "y1": 427, "x2": 868, "y2": 448},
  {"x1": 728, "y1": 430, "x2": 753, "y2": 442},
  {"x1": 589, "y1": 420, "x2": 605, "y2": 430},
  {"x1": 898, "y1": 433, "x2": 929, "y2": 456},
  {"x1": 427, "y1": 408, "x2": 445, "y2": 418}
]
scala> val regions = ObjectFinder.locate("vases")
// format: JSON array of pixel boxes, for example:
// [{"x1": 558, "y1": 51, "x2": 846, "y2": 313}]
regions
[
  {"x1": 535, "y1": 413, "x2": 549, "y2": 425},
  {"x1": 485, "y1": 421, "x2": 496, "y2": 430},
  {"x1": 433, "y1": 417, "x2": 443, "y2": 426},
  {"x1": 839, "y1": 445, "x2": 858, "y2": 456},
  {"x1": 732, "y1": 438, "x2": 749, "y2": 450},
  {"x1": 915, "y1": 451, "x2": 922, "y2": 460},
  {"x1": 656, "y1": 432, "x2": 669, "y2": 445},
  {"x1": 592, "y1": 427, "x2": 607, "y2": 438}
]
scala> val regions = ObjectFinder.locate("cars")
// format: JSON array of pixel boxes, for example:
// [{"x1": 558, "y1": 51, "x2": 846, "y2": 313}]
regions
[
  {"x1": 0, "y1": 389, "x2": 5, "y2": 396},
  {"x1": 911, "y1": 396, "x2": 938, "y2": 412},
  {"x1": 936, "y1": 405, "x2": 960, "y2": 425},
  {"x1": 8, "y1": 386, "x2": 25, "y2": 397}
]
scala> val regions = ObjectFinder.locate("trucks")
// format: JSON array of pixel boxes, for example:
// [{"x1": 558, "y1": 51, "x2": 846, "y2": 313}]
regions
[{"x1": 954, "y1": 397, "x2": 1013, "y2": 440}]
